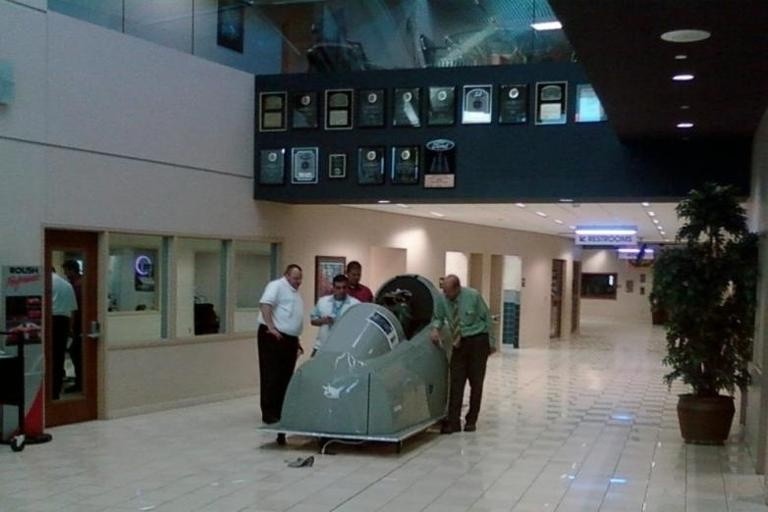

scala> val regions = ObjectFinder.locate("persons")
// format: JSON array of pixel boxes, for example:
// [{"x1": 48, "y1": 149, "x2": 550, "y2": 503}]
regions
[
  {"x1": 327, "y1": 261, "x2": 373, "y2": 303},
  {"x1": 17, "y1": 314, "x2": 41, "y2": 341},
  {"x1": 256, "y1": 264, "x2": 304, "y2": 429},
  {"x1": 429, "y1": 275, "x2": 496, "y2": 434},
  {"x1": 310, "y1": 274, "x2": 362, "y2": 359},
  {"x1": 61, "y1": 260, "x2": 82, "y2": 394},
  {"x1": 51, "y1": 265, "x2": 77, "y2": 401}
]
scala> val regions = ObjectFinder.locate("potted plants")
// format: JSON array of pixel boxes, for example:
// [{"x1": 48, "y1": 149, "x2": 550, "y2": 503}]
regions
[{"x1": 648, "y1": 181, "x2": 761, "y2": 446}]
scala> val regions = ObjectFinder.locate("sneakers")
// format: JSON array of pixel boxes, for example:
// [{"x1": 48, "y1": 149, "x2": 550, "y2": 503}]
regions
[
  {"x1": 440, "y1": 421, "x2": 461, "y2": 433},
  {"x1": 464, "y1": 421, "x2": 476, "y2": 432}
]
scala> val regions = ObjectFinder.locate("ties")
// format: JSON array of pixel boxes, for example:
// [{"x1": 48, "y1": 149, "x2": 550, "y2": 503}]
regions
[{"x1": 450, "y1": 302, "x2": 460, "y2": 348}]
